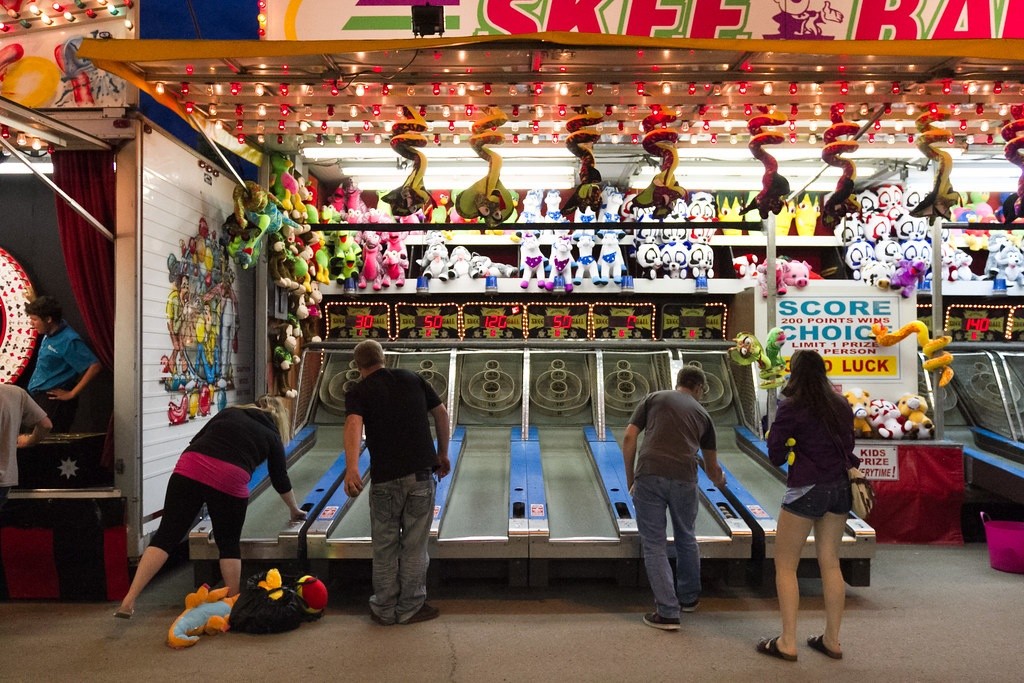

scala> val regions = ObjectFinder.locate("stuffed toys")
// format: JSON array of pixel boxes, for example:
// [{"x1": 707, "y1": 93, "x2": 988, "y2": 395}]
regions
[
  {"x1": 1001, "y1": 105, "x2": 1024, "y2": 226},
  {"x1": 844, "y1": 387, "x2": 934, "y2": 439},
  {"x1": 869, "y1": 321, "x2": 954, "y2": 387},
  {"x1": 738, "y1": 105, "x2": 790, "y2": 220},
  {"x1": 909, "y1": 103, "x2": 964, "y2": 227},
  {"x1": 256, "y1": 568, "x2": 328, "y2": 617},
  {"x1": 561, "y1": 108, "x2": 606, "y2": 211},
  {"x1": 631, "y1": 104, "x2": 687, "y2": 216},
  {"x1": 836, "y1": 183, "x2": 1024, "y2": 298},
  {"x1": 728, "y1": 325, "x2": 786, "y2": 386},
  {"x1": 381, "y1": 103, "x2": 438, "y2": 217},
  {"x1": 221, "y1": 153, "x2": 408, "y2": 397},
  {"x1": 822, "y1": 105, "x2": 860, "y2": 226},
  {"x1": 167, "y1": 583, "x2": 240, "y2": 650},
  {"x1": 716, "y1": 190, "x2": 820, "y2": 299},
  {"x1": 416, "y1": 189, "x2": 718, "y2": 292},
  {"x1": 455, "y1": 105, "x2": 514, "y2": 221}
]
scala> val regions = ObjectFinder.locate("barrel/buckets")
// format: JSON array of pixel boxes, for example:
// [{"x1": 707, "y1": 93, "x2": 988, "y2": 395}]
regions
[{"x1": 980, "y1": 511, "x2": 1024, "y2": 573}]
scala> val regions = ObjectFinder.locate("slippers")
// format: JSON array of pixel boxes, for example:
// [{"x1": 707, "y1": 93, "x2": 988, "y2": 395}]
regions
[
  {"x1": 113, "y1": 607, "x2": 136, "y2": 620},
  {"x1": 756, "y1": 636, "x2": 797, "y2": 662},
  {"x1": 806, "y1": 635, "x2": 843, "y2": 658}
]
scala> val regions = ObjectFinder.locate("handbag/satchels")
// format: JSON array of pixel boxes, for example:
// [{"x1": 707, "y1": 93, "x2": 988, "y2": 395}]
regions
[
  {"x1": 230, "y1": 570, "x2": 306, "y2": 634},
  {"x1": 847, "y1": 464, "x2": 875, "y2": 517}
]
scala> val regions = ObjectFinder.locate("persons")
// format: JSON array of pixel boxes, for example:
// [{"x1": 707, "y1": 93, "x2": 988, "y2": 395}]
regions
[
  {"x1": 24, "y1": 295, "x2": 101, "y2": 433},
  {"x1": 114, "y1": 394, "x2": 307, "y2": 618},
  {"x1": 0, "y1": 383, "x2": 53, "y2": 603},
  {"x1": 623, "y1": 367, "x2": 725, "y2": 629},
  {"x1": 344, "y1": 339, "x2": 451, "y2": 624},
  {"x1": 756, "y1": 352, "x2": 856, "y2": 661}
]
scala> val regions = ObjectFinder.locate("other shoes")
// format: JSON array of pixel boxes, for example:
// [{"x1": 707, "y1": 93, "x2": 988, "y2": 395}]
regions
[
  {"x1": 364, "y1": 597, "x2": 395, "y2": 624},
  {"x1": 400, "y1": 602, "x2": 439, "y2": 624},
  {"x1": 682, "y1": 599, "x2": 701, "y2": 611},
  {"x1": 643, "y1": 611, "x2": 681, "y2": 630}
]
